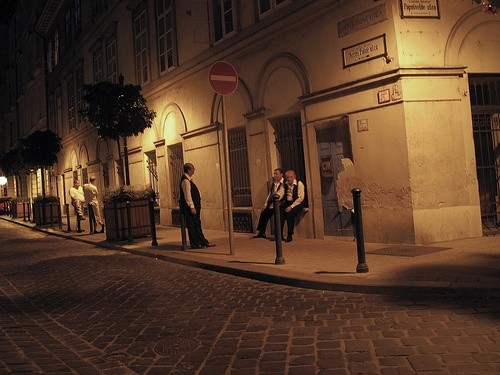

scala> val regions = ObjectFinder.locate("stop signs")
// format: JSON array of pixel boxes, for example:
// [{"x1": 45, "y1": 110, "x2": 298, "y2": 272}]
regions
[{"x1": 208, "y1": 61, "x2": 239, "y2": 96}]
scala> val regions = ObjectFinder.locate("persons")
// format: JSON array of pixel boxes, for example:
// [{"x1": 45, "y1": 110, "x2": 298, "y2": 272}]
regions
[
  {"x1": 69, "y1": 179, "x2": 86, "y2": 233},
  {"x1": 253, "y1": 168, "x2": 286, "y2": 238},
  {"x1": 178, "y1": 163, "x2": 217, "y2": 249},
  {"x1": 83, "y1": 176, "x2": 104, "y2": 233},
  {"x1": 277, "y1": 169, "x2": 304, "y2": 242}
]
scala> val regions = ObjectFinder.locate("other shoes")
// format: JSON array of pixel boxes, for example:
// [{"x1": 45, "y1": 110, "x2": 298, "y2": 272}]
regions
[
  {"x1": 203, "y1": 242, "x2": 216, "y2": 247},
  {"x1": 79, "y1": 216, "x2": 86, "y2": 220},
  {"x1": 100, "y1": 224, "x2": 104, "y2": 233},
  {"x1": 94, "y1": 229, "x2": 100, "y2": 234},
  {"x1": 253, "y1": 232, "x2": 266, "y2": 238},
  {"x1": 287, "y1": 235, "x2": 293, "y2": 242},
  {"x1": 192, "y1": 244, "x2": 206, "y2": 249}
]
